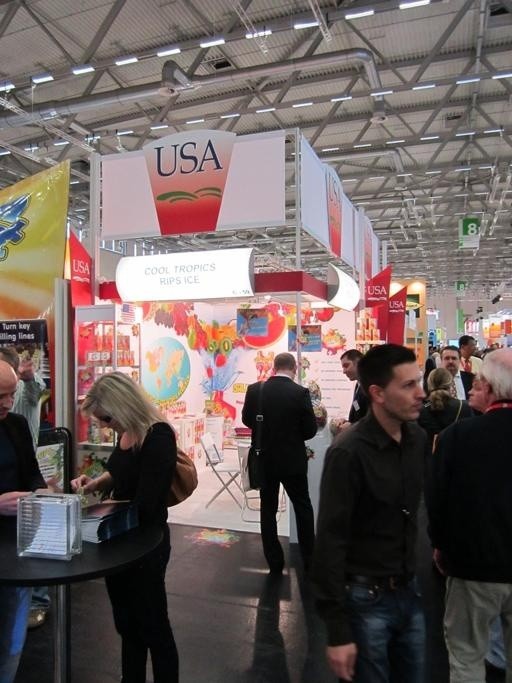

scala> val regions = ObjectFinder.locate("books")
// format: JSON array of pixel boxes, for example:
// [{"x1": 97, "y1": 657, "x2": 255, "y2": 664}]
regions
[{"x1": 79, "y1": 499, "x2": 139, "y2": 545}]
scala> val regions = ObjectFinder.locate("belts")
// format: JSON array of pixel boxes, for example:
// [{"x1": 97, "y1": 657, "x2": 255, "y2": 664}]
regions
[{"x1": 346, "y1": 573, "x2": 414, "y2": 591}]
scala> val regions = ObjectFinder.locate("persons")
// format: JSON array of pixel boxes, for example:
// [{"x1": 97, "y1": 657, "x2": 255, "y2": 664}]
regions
[
  {"x1": 0, "y1": 346, "x2": 58, "y2": 683},
  {"x1": 67, "y1": 372, "x2": 178, "y2": 683},
  {"x1": 314, "y1": 331, "x2": 512, "y2": 683},
  {"x1": 241, "y1": 351, "x2": 319, "y2": 584}
]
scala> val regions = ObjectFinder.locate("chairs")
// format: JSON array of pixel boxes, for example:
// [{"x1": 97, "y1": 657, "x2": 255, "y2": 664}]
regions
[{"x1": 200, "y1": 432, "x2": 246, "y2": 511}]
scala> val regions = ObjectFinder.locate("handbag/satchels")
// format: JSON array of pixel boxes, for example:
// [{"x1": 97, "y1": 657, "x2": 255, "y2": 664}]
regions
[
  {"x1": 247, "y1": 446, "x2": 268, "y2": 490},
  {"x1": 160, "y1": 448, "x2": 199, "y2": 510}
]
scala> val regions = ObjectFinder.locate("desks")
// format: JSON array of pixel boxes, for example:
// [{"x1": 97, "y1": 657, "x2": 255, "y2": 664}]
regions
[
  {"x1": 0, "y1": 525, "x2": 172, "y2": 682},
  {"x1": 233, "y1": 437, "x2": 287, "y2": 525}
]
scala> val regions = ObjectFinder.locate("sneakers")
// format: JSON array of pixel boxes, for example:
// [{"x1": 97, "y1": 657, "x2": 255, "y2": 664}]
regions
[{"x1": 27, "y1": 607, "x2": 47, "y2": 628}]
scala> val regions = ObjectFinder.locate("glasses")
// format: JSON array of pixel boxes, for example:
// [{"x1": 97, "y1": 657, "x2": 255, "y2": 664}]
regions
[{"x1": 98, "y1": 415, "x2": 112, "y2": 423}]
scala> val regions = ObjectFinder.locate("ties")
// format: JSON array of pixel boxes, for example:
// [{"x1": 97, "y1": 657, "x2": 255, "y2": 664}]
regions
[
  {"x1": 465, "y1": 360, "x2": 470, "y2": 373},
  {"x1": 451, "y1": 377, "x2": 457, "y2": 401}
]
